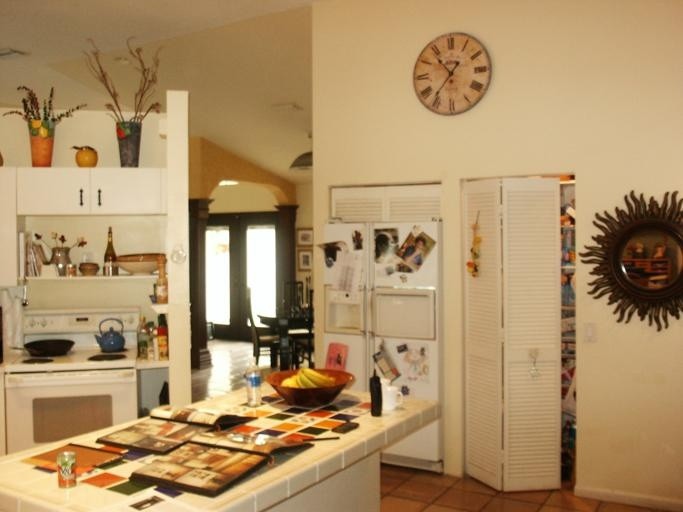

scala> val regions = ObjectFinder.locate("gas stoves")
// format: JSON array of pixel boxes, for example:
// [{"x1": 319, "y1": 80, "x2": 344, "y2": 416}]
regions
[{"x1": 22, "y1": 348, "x2": 128, "y2": 365}]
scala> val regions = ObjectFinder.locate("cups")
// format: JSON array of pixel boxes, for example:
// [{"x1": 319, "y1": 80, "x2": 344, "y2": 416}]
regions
[{"x1": 382, "y1": 386, "x2": 403, "y2": 412}]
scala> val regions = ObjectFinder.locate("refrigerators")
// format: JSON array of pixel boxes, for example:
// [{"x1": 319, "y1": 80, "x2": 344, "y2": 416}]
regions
[{"x1": 322, "y1": 221, "x2": 443, "y2": 462}]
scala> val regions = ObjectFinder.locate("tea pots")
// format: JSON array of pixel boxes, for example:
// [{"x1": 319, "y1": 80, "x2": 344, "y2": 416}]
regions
[{"x1": 94, "y1": 318, "x2": 126, "y2": 351}]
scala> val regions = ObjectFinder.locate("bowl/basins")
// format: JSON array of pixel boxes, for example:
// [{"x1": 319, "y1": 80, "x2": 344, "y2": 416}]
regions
[
  {"x1": 266, "y1": 369, "x2": 355, "y2": 406},
  {"x1": 78, "y1": 263, "x2": 99, "y2": 275},
  {"x1": 118, "y1": 253, "x2": 167, "y2": 276}
]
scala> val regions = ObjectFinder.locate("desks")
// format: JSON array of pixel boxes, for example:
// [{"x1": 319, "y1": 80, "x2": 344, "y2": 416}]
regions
[{"x1": 0, "y1": 383, "x2": 442, "y2": 512}]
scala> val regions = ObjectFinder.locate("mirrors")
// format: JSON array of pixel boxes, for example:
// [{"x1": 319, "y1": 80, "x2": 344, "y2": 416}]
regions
[{"x1": 577, "y1": 191, "x2": 682, "y2": 332}]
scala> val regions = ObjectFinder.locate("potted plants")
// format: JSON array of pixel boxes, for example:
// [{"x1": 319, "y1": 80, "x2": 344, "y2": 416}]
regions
[
  {"x1": 81, "y1": 34, "x2": 166, "y2": 169},
  {"x1": 2, "y1": 85, "x2": 90, "y2": 168}
]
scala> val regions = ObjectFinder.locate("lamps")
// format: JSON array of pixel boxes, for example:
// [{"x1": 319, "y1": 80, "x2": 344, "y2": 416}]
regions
[{"x1": 289, "y1": 131, "x2": 312, "y2": 172}]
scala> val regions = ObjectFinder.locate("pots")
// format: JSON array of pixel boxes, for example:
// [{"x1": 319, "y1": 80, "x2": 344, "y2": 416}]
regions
[{"x1": 9, "y1": 339, "x2": 75, "y2": 356}]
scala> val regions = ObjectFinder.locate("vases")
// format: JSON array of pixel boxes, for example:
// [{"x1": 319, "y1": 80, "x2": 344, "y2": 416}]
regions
[{"x1": 48, "y1": 247, "x2": 73, "y2": 276}]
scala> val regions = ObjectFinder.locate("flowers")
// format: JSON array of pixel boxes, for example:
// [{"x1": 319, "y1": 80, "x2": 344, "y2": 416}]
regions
[{"x1": 34, "y1": 231, "x2": 88, "y2": 249}]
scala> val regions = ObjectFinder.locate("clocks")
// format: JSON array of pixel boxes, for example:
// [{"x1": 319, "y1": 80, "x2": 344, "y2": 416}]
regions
[{"x1": 412, "y1": 31, "x2": 493, "y2": 116}]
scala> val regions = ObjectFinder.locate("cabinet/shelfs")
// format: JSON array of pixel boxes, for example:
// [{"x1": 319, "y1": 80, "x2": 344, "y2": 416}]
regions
[
  {"x1": 624, "y1": 257, "x2": 673, "y2": 282},
  {"x1": 5, "y1": 167, "x2": 191, "y2": 372},
  {"x1": 560, "y1": 179, "x2": 575, "y2": 487}
]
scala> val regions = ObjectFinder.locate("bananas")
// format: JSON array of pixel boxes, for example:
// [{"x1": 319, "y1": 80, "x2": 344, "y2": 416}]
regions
[{"x1": 281, "y1": 368, "x2": 336, "y2": 389}]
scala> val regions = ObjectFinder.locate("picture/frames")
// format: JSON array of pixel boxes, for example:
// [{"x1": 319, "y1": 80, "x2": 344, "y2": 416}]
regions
[
  {"x1": 297, "y1": 249, "x2": 313, "y2": 272},
  {"x1": 296, "y1": 228, "x2": 314, "y2": 247}
]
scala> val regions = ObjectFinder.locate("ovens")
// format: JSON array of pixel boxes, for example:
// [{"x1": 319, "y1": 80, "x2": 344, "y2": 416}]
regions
[{"x1": 4, "y1": 368, "x2": 138, "y2": 457}]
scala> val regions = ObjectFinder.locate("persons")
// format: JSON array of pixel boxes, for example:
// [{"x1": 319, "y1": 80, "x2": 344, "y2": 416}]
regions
[{"x1": 401, "y1": 237, "x2": 426, "y2": 268}]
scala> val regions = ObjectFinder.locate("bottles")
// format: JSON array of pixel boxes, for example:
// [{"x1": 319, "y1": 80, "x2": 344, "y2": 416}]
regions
[
  {"x1": 246, "y1": 357, "x2": 262, "y2": 406},
  {"x1": 137, "y1": 314, "x2": 168, "y2": 361},
  {"x1": 155, "y1": 255, "x2": 168, "y2": 304},
  {"x1": 103, "y1": 227, "x2": 118, "y2": 276},
  {"x1": 369, "y1": 367, "x2": 382, "y2": 416}
]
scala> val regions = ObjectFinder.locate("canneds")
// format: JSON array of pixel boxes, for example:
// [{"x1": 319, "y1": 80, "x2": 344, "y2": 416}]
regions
[{"x1": 56, "y1": 451, "x2": 76, "y2": 488}]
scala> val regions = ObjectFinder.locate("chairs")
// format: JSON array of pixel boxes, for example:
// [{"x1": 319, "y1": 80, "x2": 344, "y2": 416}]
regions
[{"x1": 245, "y1": 287, "x2": 315, "y2": 370}]
